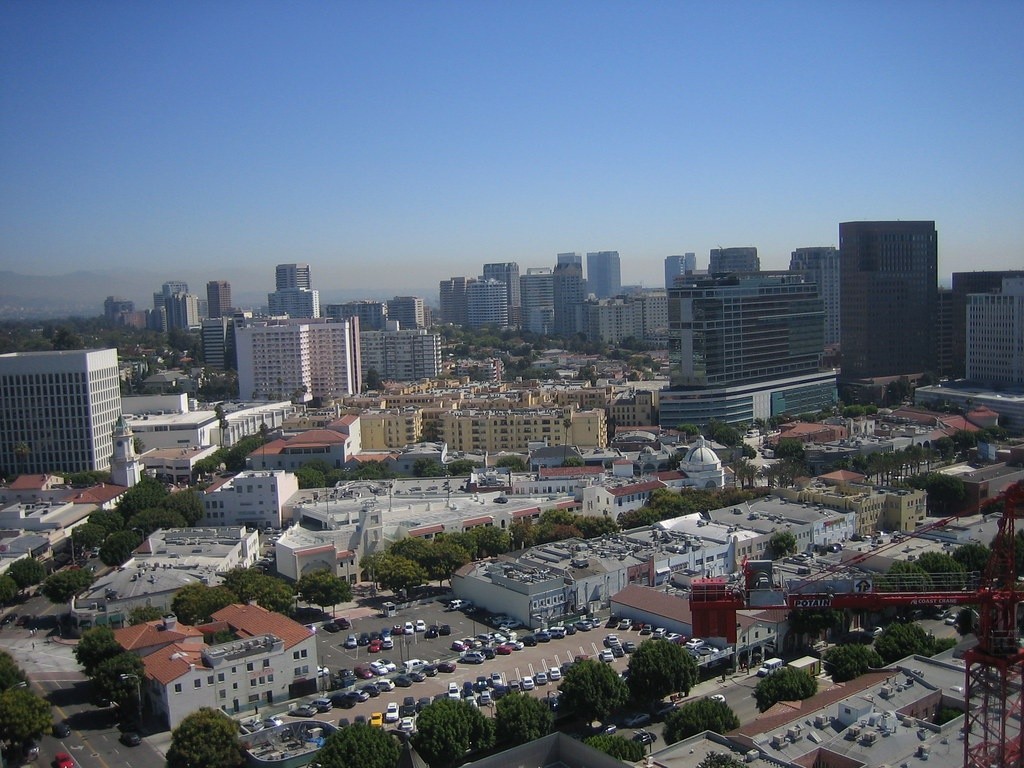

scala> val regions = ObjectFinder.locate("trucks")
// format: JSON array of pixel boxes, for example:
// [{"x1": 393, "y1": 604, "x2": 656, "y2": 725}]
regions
[{"x1": 757, "y1": 658, "x2": 783, "y2": 679}]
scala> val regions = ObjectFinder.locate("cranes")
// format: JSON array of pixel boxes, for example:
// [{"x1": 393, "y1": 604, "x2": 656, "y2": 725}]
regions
[{"x1": 686, "y1": 474, "x2": 1024, "y2": 768}]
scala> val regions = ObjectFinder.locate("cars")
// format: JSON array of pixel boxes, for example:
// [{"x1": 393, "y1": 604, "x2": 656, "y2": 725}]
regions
[
  {"x1": 1, "y1": 613, "x2": 18, "y2": 625},
  {"x1": 120, "y1": 732, "x2": 142, "y2": 747},
  {"x1": 55, "y1": 751, "x2": 74, "y2": 768},
  {"x1": 652, "y1": 627, "x2": 668, "y2": 640},
  {"x1": 640, "y1": 623, "x2": 656, "y2": 635},
  {"x1": 240, "y1": 593, "x2": 724, "y2": 734},
  {"x1": 934, "y1": 608, "x2": 951, "y2": 621},
  {"x1": 792, "y1": 552, "x2": 811, "y2": 562},
  {"x1": 632, "y1": 732, "x2": 658, "y2": 747},
  {"x1": 52, "y1": 724, "x2": 71, "y2": 739},
  {"x1": 665, "y1": 632, "x2": 683, "y2": 644},
  {"x1": 652, "y1": 702, "x2": 680, "y2": 720},
  {"x1": 623, "y1": 711, "x2": 651, "y2": 728},
  {"x1": 709, "y1": 694, "x2": 726, "y2": 704},
  {"x1": 946, "y1": 612, "x2": 959, "y2": 626}
]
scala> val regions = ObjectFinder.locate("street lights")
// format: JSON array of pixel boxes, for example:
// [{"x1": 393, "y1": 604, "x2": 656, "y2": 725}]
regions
[
  {"x1": 120, "y1": 673, "x2": 144, "y2": 727},
  {"x1": 133, "y1": 526, "x2": 144, "y2": 543}
]
